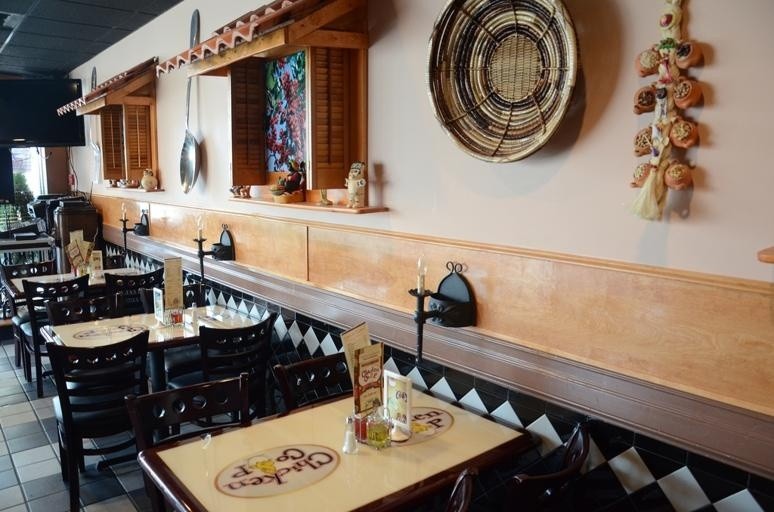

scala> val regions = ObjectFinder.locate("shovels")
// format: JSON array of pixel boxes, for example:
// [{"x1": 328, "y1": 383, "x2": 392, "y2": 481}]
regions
[
  {"x1": 177, "y1": 7, "x2": 205, "y2": 196},
  {"x1": 89, "y1": 66, "x2": 100, "y2": 186}
]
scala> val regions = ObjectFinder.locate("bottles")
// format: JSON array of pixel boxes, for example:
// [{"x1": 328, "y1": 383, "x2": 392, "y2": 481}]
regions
[
  {"x1": 192, "y1": 301, "x2": 198, "y2": 325},
  {"x1": 341, "y1": 416, "x2": 359, "y2": 455}
]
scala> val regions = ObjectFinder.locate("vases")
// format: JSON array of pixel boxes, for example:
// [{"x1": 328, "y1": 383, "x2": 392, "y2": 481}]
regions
[{"x1": 141, "y1": 169, "x2": 158, "y2": 191}]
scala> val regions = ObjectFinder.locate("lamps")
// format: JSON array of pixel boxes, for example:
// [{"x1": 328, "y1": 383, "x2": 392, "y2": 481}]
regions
[
  {"x1": 191, "y1": 214, "x2": 236, "y2": 284},
  {"x1": 118, "y1": 201, "x2": 150, "y2": 252}
]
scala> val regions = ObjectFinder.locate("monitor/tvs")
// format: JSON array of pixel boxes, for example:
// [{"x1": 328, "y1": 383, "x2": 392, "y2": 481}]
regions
[{"x1": 0, "y1": 74, "x2": 87, "y2": 148}]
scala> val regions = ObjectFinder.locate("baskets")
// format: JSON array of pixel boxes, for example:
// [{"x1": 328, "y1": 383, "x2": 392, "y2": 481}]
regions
[{"x1": 427, "y1": 0, "x2": 579, "y2": 166}]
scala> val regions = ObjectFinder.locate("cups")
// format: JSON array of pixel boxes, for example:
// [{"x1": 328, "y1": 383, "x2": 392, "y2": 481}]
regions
[
  {"x1": 170, "y1": 306, "x2": 184, "y2": 323},
  {"x1": 366, "y1": 415, "x2": 392, "y2": 449}
]
